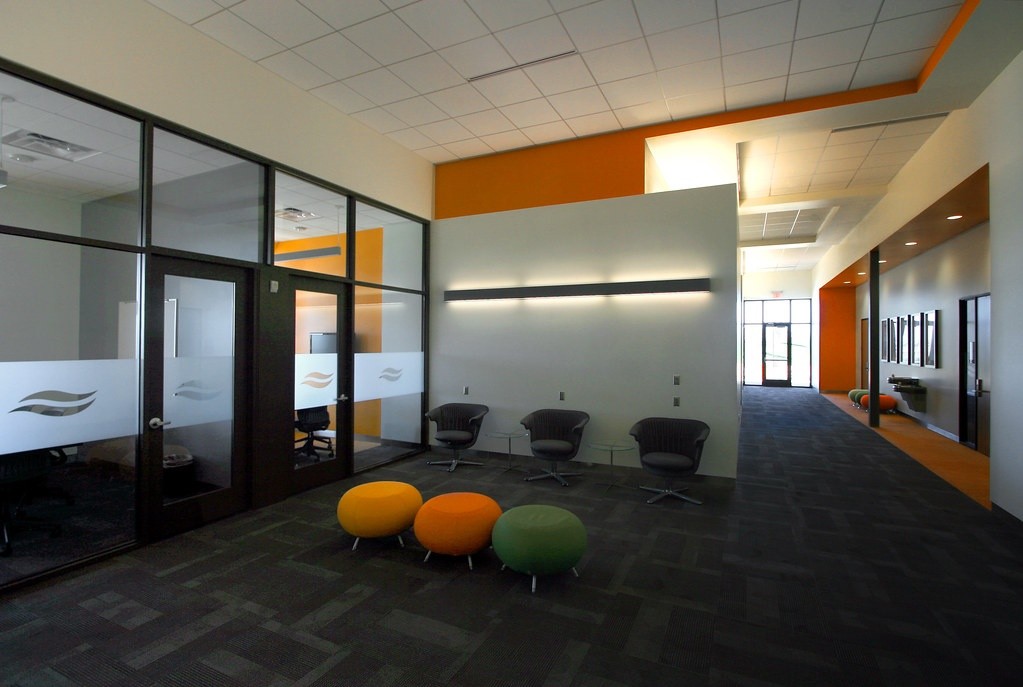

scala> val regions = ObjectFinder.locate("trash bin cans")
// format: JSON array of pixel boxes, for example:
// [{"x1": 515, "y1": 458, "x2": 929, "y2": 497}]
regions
[{"x1": 162, "y1": 453, "x2": 193, "y2": 500}]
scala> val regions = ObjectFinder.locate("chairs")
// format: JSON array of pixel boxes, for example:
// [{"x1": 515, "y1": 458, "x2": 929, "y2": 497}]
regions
[
  {"x1": 426, "y1": 402, "x2": 489, "y2": 471},
  {"x1": 629, "y1": 417, "x2": 710, "y2": 505},
  {"x1": 521, "y1": 408, "x2": 590, "y2": 487},
  {"x1": 294, "y1": 404, "x2": 334, "y2": 463}
]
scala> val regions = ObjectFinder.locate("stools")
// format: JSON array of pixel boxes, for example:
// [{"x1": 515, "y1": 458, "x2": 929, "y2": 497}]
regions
[
  {"x1": 861, "y1": 395, "x2": 897, "y2": 415},
  {"x1": 413, "y1": 493, "x2": 502, "y2": 571},
  {"x1": 490, "y1": 505, "x2": 587, "y2": 593},
  {"x1": 337, "y1": 479, "x2": 423, "y2": 552},
  {"x1": 855, "y1": 392, "x2": 886, "y2": 409},
  {"x1": 848, "y1": 389, "x2": 870, "y2": 406}
]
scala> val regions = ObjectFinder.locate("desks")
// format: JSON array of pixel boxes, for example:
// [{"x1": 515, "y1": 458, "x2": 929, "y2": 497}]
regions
[
  {"x1": 588, "y1": 436, "x2": 634, "y2": 495},
  {"x1": 486, "y1": 430, "x2": 528, "y2": 475}
]
